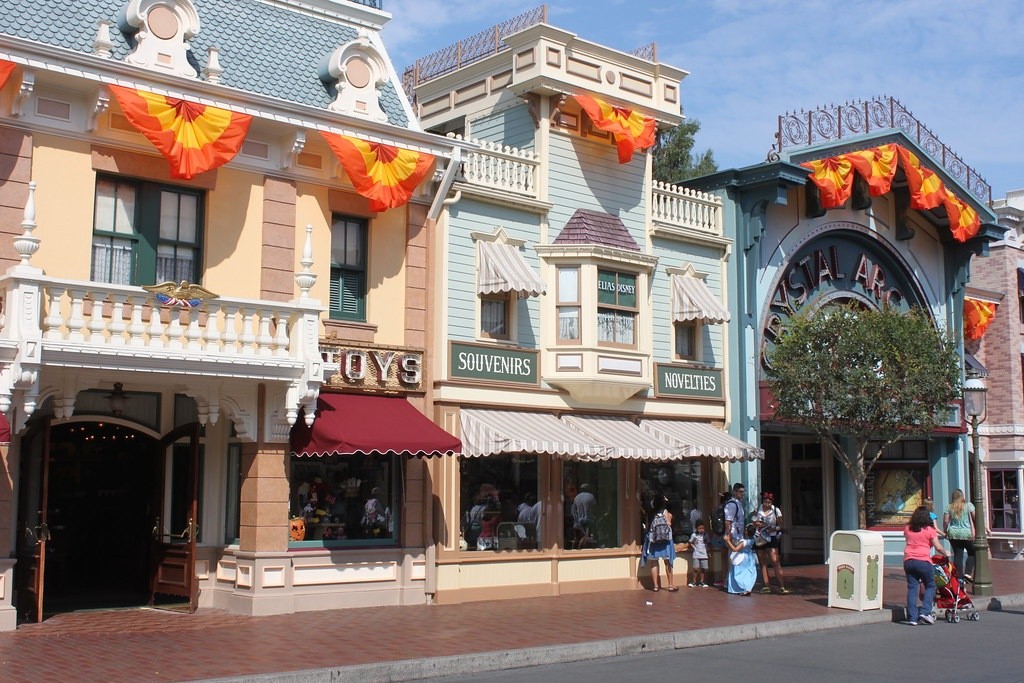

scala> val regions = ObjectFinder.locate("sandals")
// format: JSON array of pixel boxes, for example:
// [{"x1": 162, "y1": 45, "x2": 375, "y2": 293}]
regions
[
  {"x1": 740, "y1": 591, "x2": 750, "y2": 596},
  {"x1": 653, "y1": 586, "x2": 659, "y2": 592},
  {"x1": 669, "y1": 586, "x2": 678, "y2": 592}
]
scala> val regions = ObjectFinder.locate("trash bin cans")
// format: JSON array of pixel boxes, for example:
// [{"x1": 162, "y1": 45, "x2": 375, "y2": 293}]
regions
[{"x1": 828, "y1": 530, "x2": 884, "y2": 612}]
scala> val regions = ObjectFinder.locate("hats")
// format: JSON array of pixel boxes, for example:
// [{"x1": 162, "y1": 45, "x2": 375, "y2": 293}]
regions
[
  {"x1": 371, "y1": 488, "x2": 385, "y2": 495},
  {"x1": 762, "y1": 493, "x2": 773, "y2": 501}
]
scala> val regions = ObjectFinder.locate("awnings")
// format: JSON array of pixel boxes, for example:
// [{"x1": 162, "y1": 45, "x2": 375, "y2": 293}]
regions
[
  {"x1": 635, "y1": 418, "x2": 765, "y2": 463},
  {"x1": 479, "y1": 240, "x2": 546, "y2": 299},
  {"x1": 0, "y1": 412, "x2": 10, "y2": 444},
  {"x1": 674, "y1": 277, "x2": 731, "y2": 325},
  {"x1": 290, "y1": 391, "x2": 462, "y2": 459},
  {"x1": 965, "y1": 349, "x2": 989, "y2": 377},
  {"x1": 553, "y1": 414, "x2": 682, "y2": 462},
  {"x1": 459, "y1": 408, "x2": 607, "y2": 459}
]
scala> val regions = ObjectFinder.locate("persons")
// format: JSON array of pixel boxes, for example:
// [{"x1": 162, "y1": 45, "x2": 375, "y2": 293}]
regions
[
  {"x1": 461, "y1": 480, "x2": 598, "y2": 549},
  {"x1": 298, "y1": 477, "x2": 330, "y2": 516},
  {"x1": 723, "y1": 523, "x2": 757, "y2": 595},
  {"x1": 903, "y1": 506, "x2": 952, "y2": 625},
  {"x1": 362, "y1": 487, "x2": 386, "y2": 538},
  {"x1": 646, "y1": 497, "x2": 678, "y2": 592},
  {"x1": 688, "y1": 519, "x2": 711, "y2": 587},
  {"x1": 943, "y1": 489, "x2": 975, "y2": 582},
  {"x1": 724, "y1": 483, "x2": 745, "y2": 558},
  {"x1": 923, "y1": 499, "x2": 945, "y2": 560},
  {"x1": 752, "y1": 492, "x2": 789, "y2": 594}
]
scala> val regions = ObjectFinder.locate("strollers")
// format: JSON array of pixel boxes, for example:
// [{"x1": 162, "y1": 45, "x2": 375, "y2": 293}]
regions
[{"x1": 919, "y1": 554, "x2": 980, "y2": 624}]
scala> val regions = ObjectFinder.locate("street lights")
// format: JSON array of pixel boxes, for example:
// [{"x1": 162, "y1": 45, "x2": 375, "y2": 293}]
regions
[{"x1": 962, "y1": 365, "x2": 995, "y2": 595}]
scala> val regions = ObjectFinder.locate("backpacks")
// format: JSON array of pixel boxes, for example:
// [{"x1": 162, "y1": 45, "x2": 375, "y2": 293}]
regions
[
  {"x1": 712, "y1": 501, "x2": 738, "y2": 536},
  {"x1": 650, "y1": 512, "x2": 669, "y2": 544}
]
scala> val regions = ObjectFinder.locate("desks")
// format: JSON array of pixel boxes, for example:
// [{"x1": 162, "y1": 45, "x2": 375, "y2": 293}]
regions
[{"x1": 307, "y1": 522, "x2": 345, "y2": 540}]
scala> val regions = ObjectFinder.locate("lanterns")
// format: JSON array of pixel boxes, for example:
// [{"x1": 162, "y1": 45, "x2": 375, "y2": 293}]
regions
[{"x1": 291, "y1": 515, "x2": 305, "y2": 541}]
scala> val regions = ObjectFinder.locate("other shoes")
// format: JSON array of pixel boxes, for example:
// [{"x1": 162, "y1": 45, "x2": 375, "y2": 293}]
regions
[
  {"x1": 760, "y1": 586, "x2": 772, "y2": 593},
  {"x1": 777, "y1": 587, "x2": 790, "y2": 595},
  {"x1": 699, "y1": 583, "x2": 707, "y2": 587},
  {"x1": 910, "y1": 621, "x2": 917, "y2": 626},
  {"x1": 963, "y1": 574, "x2": 973, "y2": 582},
  {"x1": 920, "y1": 614, "x2": 935, "y2": 625},
  {"x1": 688, "y1": 582, "x2": 695, "y2": 587}
]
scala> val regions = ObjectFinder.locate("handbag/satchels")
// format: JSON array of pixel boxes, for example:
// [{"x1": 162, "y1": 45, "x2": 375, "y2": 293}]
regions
[
  {"x1": 714, "y1": 578, "x2": 727, "y2": 586},
  {"x1": 465, "y1": 526, "x2": 479, "y2": 546},
  {"x1": 756, "y1": 536, "x2": 768, "y2": 546}
]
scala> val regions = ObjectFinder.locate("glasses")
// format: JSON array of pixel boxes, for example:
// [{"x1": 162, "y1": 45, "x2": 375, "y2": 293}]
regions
[{"x1": 530, "y1": 498, "x2": 537, "y2": 502}]
